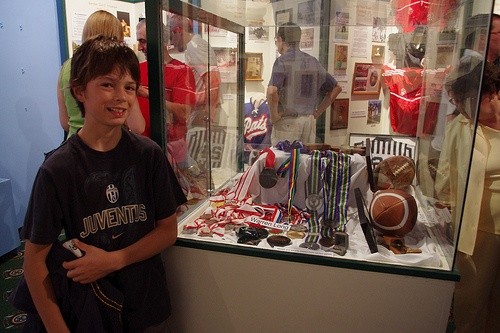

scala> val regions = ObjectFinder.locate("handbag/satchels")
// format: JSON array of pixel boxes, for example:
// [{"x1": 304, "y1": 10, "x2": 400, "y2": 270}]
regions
[{"x1": 7, "y1": 236, "x2": 89, "y2": 322}]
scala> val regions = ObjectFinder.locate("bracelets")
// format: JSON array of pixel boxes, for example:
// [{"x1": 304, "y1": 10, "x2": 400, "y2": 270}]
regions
[{"x1": 315, "y1": 107, "x2": 322, "y2": 114}]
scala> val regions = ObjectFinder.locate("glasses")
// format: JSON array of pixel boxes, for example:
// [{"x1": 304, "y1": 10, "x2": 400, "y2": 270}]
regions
[{"x1": 449, "y1": 96, "x2": 478, "y2": 105}]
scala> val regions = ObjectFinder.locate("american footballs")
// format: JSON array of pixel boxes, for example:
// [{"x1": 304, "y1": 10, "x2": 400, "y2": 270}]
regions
[
  {"x1": 373, "y1": 155, "x2": 416, "y2": 191},
  {"x1": 368, "y1": 189, "x2": 418, "y2": 236}
]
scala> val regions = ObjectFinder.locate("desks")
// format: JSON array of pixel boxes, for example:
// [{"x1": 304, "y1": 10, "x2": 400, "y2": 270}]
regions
[{"x1": 0, "y1": 178, "x2": 22, "y2": 256}]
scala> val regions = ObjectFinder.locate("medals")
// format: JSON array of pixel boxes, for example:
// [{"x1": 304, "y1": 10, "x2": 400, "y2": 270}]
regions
[{"x1": 181, "y1": 168, "x2": 334, "y2": 249}]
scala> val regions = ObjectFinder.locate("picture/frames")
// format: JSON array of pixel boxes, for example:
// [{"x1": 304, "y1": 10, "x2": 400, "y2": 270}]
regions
[
  {"x1": 275, "y1": 8, "x2": 293, "y2": 39},
  {"x1": 354, "y1": 188, "x2": 378, "y2": 253},
  {"x1": 365, "y1": 138, "x2": 379, "y2": 191},
  {"x1": 329, "y1": 98, "x2": 350, "y2": 130},
  {"x1": 244, "y1": 53, "x2": 263, "y2": 82},
  {"x1": 350, "y1": 63, "x2": 385, "y2": 97}
]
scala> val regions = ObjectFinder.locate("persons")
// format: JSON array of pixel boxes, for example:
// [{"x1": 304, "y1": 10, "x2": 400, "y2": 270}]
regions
[
  {"x1": 435, "y1": 12, "x2": 500, "y2": 333},
  {"x1": 57, "y1": 9, "x2": 145, "y2": 142},
  {"x1": 136, "y1": 19, "x2": 196, "y2": 217},
  {"x1": 169, "y1": 15, "x2": 222, "y2": 197},
  {"x1": 20, "y1": 33, "x2": 188, "y2": 333},
  {"x1": 267, "y1": 22, "x2": 341, "y2": 143}
]
